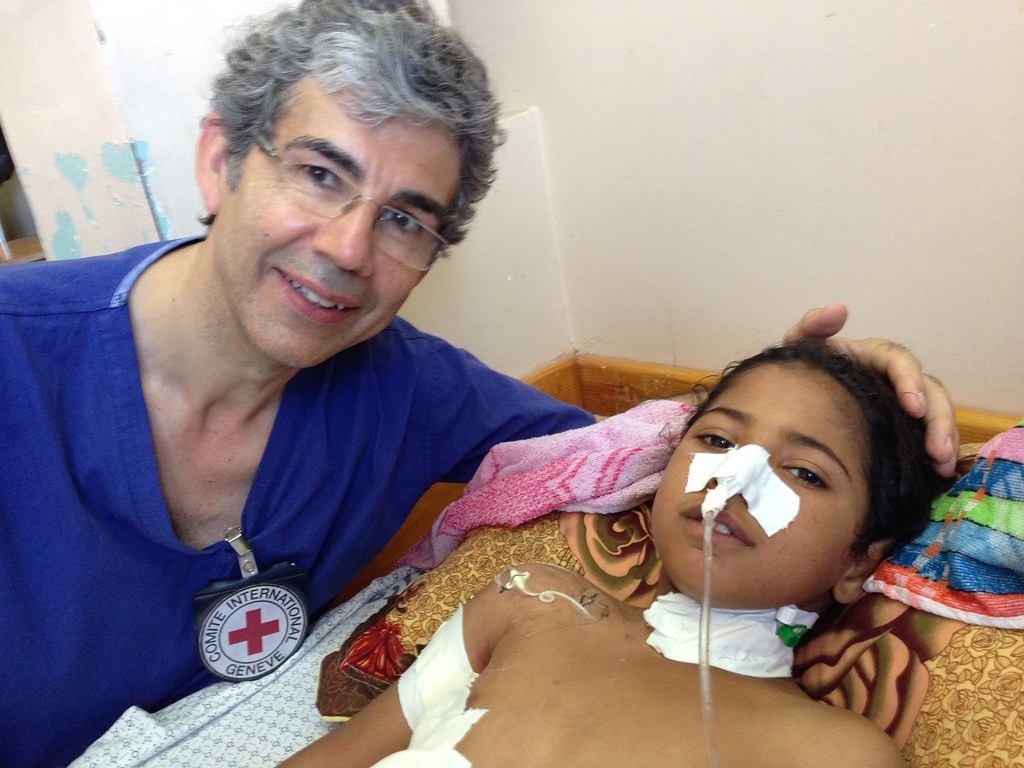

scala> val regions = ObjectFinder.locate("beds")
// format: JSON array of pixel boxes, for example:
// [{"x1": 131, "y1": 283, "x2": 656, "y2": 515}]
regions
[{"x1": 66, "y1": 351, "x2": 1024, "y2": 768}]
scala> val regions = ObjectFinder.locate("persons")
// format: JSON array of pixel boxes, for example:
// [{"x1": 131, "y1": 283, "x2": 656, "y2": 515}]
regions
[
  {"x1": 272, "y1": 342, "x2": 930, "y2": 768},
  {"x1": 0, "y1": 0, "x2": 958, "y2": 768}
]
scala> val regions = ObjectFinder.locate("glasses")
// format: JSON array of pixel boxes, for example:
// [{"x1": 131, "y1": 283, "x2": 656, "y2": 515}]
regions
[{"x1": 256, "y1": 137, "x2": 448, "y2": 271}]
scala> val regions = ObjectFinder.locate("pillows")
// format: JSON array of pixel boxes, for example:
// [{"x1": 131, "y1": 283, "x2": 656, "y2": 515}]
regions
[{"x1": 318, "y1": 499, "x2": 1024, "y2": 768}]
